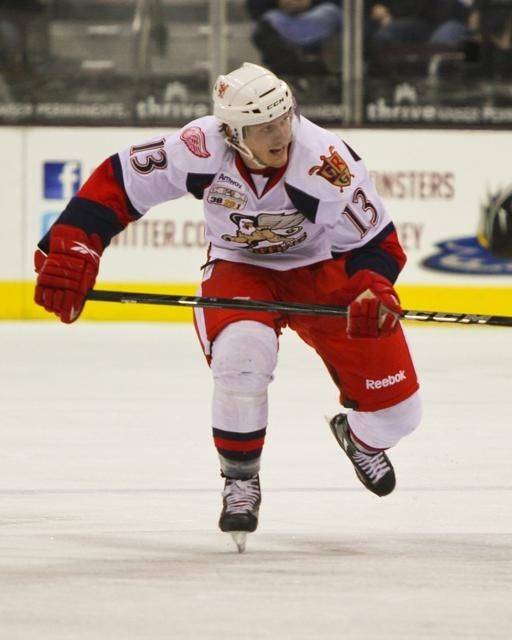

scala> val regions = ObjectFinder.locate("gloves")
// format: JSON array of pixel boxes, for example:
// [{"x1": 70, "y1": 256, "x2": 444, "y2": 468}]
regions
[
  {"x1": 329, "y1": 270, "x2": 403, "y2": 338},
  {"x1": 33, "y1": 224, "x2": 103, "y2": 323}
]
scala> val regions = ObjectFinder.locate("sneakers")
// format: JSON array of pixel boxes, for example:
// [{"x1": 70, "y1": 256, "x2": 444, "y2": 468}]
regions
[
  {"x1": 218, "y1": 468, "x2": 260, "y2": 533},
  {"x1": 329, "y1": 413, "x2": 395, "y2": 497}
]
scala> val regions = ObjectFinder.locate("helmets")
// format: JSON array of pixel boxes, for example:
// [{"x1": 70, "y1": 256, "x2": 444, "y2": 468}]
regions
[{"x1": 211, "y1": 62, "x2": 300, "y2": 160}]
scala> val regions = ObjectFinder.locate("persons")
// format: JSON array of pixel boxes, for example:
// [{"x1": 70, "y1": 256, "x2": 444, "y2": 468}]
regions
[
  {"x1": 240, "y1": 1, "x2": 512, "y2": 118},
  {"x1": 28, "y1": 60, "x2": 424, "y2": 538}
]
